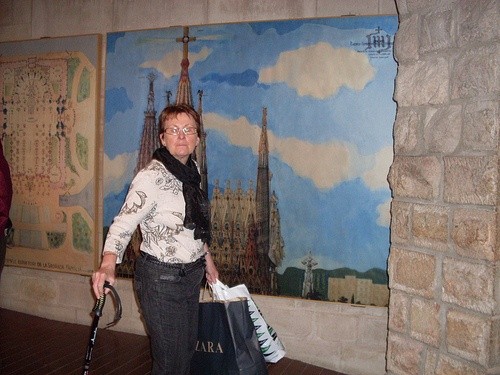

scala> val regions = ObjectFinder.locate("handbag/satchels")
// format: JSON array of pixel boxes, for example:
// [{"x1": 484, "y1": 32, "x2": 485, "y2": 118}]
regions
[
  {"x1": 207, "y1": 279, "x2": 286, "y2": 363},
  {"x1": 191, "y1": 278, "x2": 269, "y2": 375}
]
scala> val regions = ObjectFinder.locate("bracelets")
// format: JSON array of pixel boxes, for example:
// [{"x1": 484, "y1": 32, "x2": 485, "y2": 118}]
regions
[{"x1": 204, "y1": 250, "x2": 212, "y2": 256}]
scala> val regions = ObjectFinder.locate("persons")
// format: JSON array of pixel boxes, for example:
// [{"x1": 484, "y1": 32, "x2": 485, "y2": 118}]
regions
[
  {"x1": 0, "y1": 140, "x2": 13, "y2": 278},
  {"x1": 91, "y1": 103, "x2": 219, "y2": 374}
]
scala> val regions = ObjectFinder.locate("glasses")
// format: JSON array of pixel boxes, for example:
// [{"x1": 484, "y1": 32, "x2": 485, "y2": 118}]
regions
[{"x1": 163, "y1": 127, "x2": 199, "y2": 135}]
